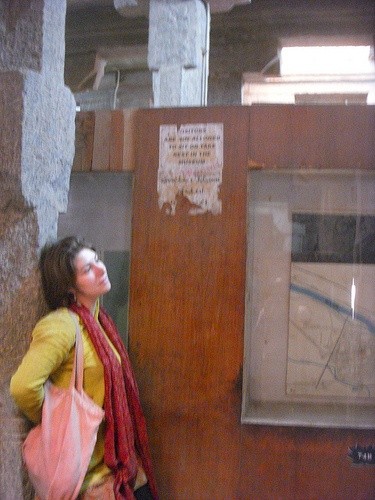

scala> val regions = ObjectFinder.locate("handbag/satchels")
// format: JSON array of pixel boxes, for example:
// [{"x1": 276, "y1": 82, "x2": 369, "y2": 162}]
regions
[{"x1": 19, "y1": 316, "x2": 105, "y2": 500}]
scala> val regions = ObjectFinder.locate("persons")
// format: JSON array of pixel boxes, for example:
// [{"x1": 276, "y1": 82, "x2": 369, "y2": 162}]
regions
[{"x1": 10, "y1": 237, "x2": 158, "y2": 500}]
